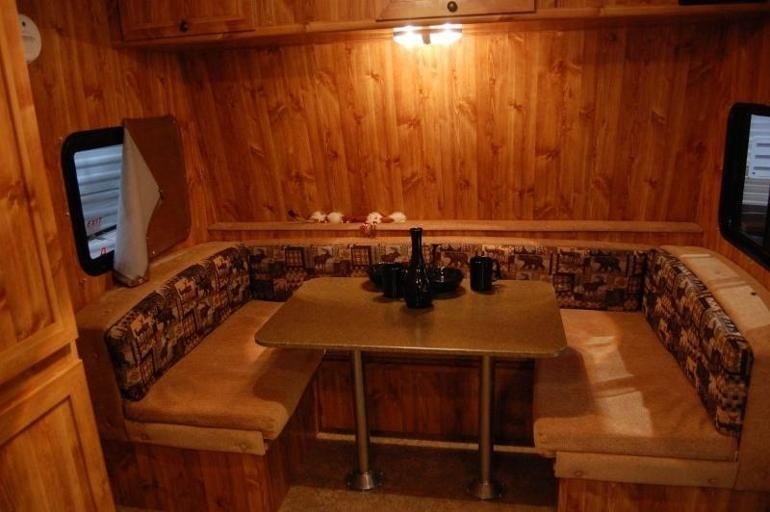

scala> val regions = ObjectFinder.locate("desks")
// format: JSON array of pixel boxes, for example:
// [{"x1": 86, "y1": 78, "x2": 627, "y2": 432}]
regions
[{"x1": 254, "y1": 275, "x2": 568, "y2": 499}]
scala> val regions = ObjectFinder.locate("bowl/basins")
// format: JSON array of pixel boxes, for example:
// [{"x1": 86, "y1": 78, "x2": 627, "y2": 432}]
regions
[
  {"x1": 367, "y1": 262, "x2": 405, "y2": 288},
  {"x1": 427, "y1": 268, "x2": 463, "y2": 291}
]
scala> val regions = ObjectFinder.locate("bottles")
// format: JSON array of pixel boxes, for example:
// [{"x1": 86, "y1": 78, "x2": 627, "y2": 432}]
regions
[{"x1": 403, "y1": 226, "x2": 434, "y2": 308}]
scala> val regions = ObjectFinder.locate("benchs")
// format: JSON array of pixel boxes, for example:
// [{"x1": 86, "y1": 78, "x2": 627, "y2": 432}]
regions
[{"x1": 73, "y1": 237, "x2": 769, "y2": 512}]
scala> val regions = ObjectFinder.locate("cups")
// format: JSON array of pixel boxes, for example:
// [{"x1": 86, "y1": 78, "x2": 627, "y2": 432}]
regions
[
  {"x1": 468, "y1": 256, "x2": 501, "y2": 293},
  {"x1": 383, "y1": 271, "x2": 407, "y2": 297}
]
